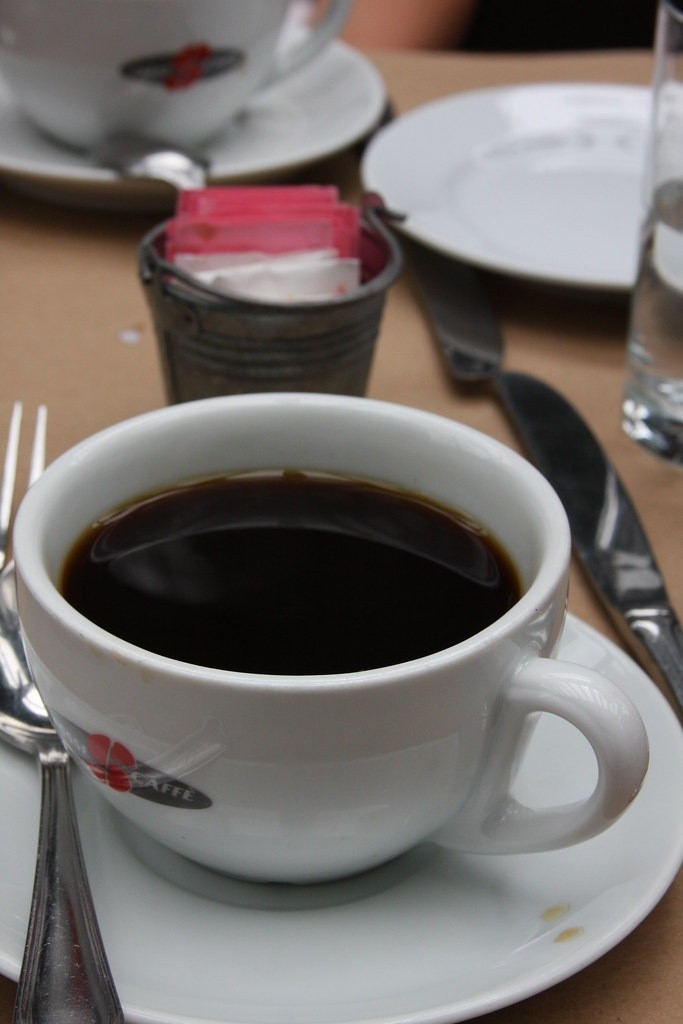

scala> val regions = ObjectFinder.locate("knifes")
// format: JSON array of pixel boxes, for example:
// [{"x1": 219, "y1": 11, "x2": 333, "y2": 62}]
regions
[
  {"x1": 492, "y1": 370, "x2": 683, "y2": 723},
  {"x1": 358, "y1": 99, "x2": 502, "y2": 399}
]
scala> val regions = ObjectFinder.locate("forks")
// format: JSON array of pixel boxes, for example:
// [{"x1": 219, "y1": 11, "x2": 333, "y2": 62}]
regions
[{"x1": 0, "y1": 404, "x2": 48, "y2": 548}]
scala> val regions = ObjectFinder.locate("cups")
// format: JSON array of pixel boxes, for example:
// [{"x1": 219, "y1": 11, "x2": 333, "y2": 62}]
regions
[
  {"x1": 623, "y1": 0, "x2": 683, "y2": 464},
  {"x1": 12, "y1": 391, "x2": 649, "y2": 883},
  {"x1": 0, "y1": 0, "x2": 352, "y2": 150},
  {"x1": 139, "y1": 205, "x2": 401, "y2": 406}
]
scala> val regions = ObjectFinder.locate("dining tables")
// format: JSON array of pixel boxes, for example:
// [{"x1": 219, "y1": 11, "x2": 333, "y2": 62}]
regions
[{"x1": 1, "y1": 52, "x2": 683, "y2": 1024}]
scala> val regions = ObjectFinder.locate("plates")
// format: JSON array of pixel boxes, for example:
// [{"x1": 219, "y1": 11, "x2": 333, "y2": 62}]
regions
[
  {"x1": 360, "y1": 82, "x2": 683, "y2": 291},
  {"x1": 0, "y1": 24, "x2": 385, "y2": 207},
  {"x1": 0, "y1": 616, "x2": 681, "y2": 1023}
]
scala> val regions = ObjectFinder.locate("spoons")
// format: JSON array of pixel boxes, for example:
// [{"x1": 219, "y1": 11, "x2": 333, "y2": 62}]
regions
[
  {"x1": 0, "y1": 555, "x2": 125, "y2": 1024},
  {"x1": 54, "y1": 138, "x2": 206, "y2": 194}
]
